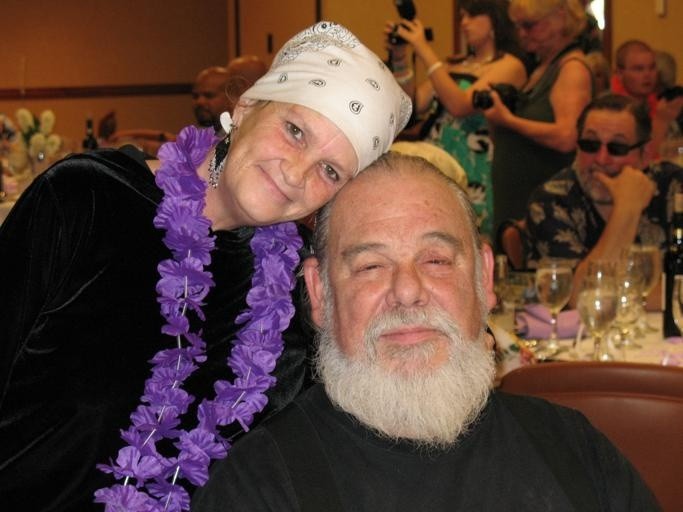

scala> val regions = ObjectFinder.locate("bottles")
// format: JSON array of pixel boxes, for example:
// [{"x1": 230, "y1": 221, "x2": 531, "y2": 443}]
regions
[{"x1": 660, "y1": 192, "x2": 683, "y2": 340}]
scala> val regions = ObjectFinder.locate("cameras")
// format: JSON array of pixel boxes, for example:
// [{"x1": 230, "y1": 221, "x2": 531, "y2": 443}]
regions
[
  {"x1": 386, "y1": 0, "x2": 434, "y2": 44},
  {"x1": 472, "y1": 83, "x2": 517, "y2": 115}
]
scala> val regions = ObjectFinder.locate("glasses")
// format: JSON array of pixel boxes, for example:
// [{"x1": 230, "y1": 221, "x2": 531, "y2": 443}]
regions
[
  {"x1": 515, "y1": 2, "x2": 567, "y2": 31},
  {"x1": 573, "y1": 134, "x2": 645, "y2": 160}
]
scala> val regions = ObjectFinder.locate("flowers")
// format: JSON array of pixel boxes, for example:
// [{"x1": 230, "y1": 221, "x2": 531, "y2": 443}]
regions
[{"x1": 1, "y1": 106, "x2": 64, "y2": 185}]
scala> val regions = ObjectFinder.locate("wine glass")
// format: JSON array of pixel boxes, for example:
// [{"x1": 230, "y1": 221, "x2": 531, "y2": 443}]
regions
[
  {"x1": 528, "y1": 260, "x2": 576, "y2": 361},
  {"x1": 574, "y1": 247, "x2": 660, "y2": 363},
  {"x1": 496, "y1": 272, "x2": 530, "y2": 355}
]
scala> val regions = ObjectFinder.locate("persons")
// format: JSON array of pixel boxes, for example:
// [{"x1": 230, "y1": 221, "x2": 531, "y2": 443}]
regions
[
  {"x1": 184, "y1": 149, "x2": 657, "y2": 512},
  {"x1": 1, "y1": 21, "x2": 499, "y2": 512}
]
